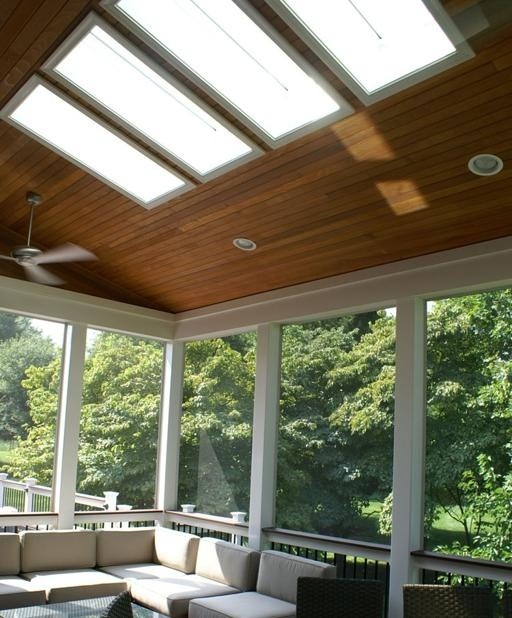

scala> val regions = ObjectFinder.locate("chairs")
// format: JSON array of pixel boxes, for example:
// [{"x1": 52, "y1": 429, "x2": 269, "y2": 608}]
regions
[{"x1": 1, "y1": 525, "x2": 492, "y2": 618}]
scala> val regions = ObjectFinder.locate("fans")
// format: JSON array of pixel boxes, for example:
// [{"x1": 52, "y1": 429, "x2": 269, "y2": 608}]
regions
[{"x1": 0, "y1": 191, "x2": 100, "y2": 288}]
